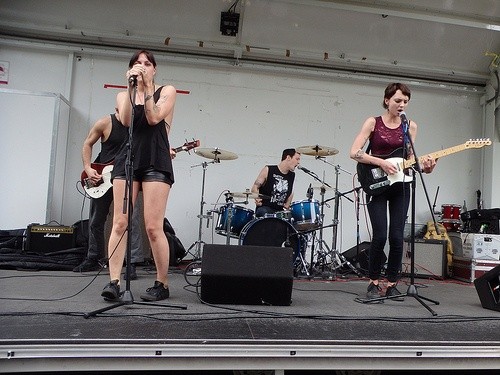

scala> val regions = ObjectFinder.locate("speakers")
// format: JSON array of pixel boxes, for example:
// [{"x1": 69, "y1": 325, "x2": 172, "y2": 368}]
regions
[
  {"x1": 400, "y1": 238, "x2": 447, "y2": 279},
  {"x1": 200, "y1": 243, "x2": 294, "y2": 306},
  {"x1": 337, "y1": 241, "x2": 387, "y2": 275},
  {"x1": 30, "y1": 225, "x2": 75, "y2": 253},
  {"x1": 474, "y1": 264, "x2": 500, "y2": 312}
]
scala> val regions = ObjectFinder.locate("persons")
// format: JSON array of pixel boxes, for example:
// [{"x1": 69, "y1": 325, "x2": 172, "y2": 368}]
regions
[
  {"x1": 101, "y1": 49, "x2": 175, "y2": 300},
  {"x1": 252, "y1": 148, "x2": 307, "y2": 271},
  {"x1": 351, "y1": 83, "x2": 439, "y2": 301},
  {"x1": 73, "y1": 102, "x2": 145, "y2": 280}
]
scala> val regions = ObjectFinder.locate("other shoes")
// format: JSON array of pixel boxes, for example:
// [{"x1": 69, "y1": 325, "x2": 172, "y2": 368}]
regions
[
  {"x1": 73, "y1": 261, "x2": 99, "y2": 272},
  {"x1": 124, "y1": 268, "x2": 138, "y2": 280}
]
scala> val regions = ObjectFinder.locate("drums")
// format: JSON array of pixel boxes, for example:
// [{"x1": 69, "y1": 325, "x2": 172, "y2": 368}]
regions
[
  {"x1": 443, "y1": 202, "x2": 461, "y2": 230},
  {"x1": 240, "y1": 214, "x2": 300, "y2": 268},
  {"x1": 215, "y1": 205, "x2": 256, "y2": 240},
  {"x1": 291, "y1": 199, "x2": 322, "y2": 229}
]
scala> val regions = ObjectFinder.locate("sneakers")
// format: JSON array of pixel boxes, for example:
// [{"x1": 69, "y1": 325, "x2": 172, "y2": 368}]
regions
[
  {"x1": 101, "y1": 279, "x2": 120, "y2": 301},
  {"x1": 386, "y1": 284, "x2": 405, "y2": 301},
  {"x1": 367, "y1": 281, "x2": 382, "y2": 297},
  {"x1": 140, "y1": 281, "x2": 169, "y2": 301}
]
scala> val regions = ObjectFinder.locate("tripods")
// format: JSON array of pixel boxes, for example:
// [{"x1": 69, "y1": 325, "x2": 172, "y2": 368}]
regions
[
  {"x1": 175, "y1": 157, "x2": 220, "y2": 263},
  {"x1": 359, "y1": 124, "x2": 440, "y2": 317},
  {"x1": 293, "y1": 154, "x2": 363, "y2": 279}
]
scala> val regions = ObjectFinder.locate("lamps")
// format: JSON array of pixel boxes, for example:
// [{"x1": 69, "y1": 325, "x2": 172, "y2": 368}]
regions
[{"x1": 219, "y1": 1, "x2": 242, "y2": 38}]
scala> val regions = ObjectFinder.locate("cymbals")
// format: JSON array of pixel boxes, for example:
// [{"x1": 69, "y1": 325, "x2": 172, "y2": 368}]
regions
[
  {"x1": 314, "y1": 186, "x2": 334, "y2": 191},
  {"x1": 295, "y1": 145, "x2": 339, "y2": 157},
  {"x1": 193, "y1": 147, "x2": 239, "y2": 161},
  {"x1": 230, "y1": 192, "x2": 265, "y2": 198}
]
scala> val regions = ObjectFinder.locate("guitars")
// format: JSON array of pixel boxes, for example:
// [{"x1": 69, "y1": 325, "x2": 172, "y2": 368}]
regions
[
  {"x1": 80, "y1": 138, "x2": 199, "y2": 198},
  {"x1": 356, "y1": 138, "x2": 492, "y2": 197}
]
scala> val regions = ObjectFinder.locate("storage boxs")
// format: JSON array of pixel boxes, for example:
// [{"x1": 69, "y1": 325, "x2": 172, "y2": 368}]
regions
[
  {"x1": 461, "y1": 232, "x2": 500, "y2": 260},
  {"x1": 451, "y1": 255, "x2": 500, "y2": 284}
]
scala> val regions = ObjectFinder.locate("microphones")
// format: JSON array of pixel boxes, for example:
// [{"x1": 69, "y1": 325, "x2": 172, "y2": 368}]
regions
[
  {"x1": 281, "y1": 241, "x2": 290, "y2": 247},
  {"x1": 297, "y1": 165, "x2": 310, "y2": 173},
  {"x1": 129, "y1": 75, "x2": 137, "y2": 83},
  {"x1": 398, "y1": 111, "x2": 409, "y2": 129}
]
